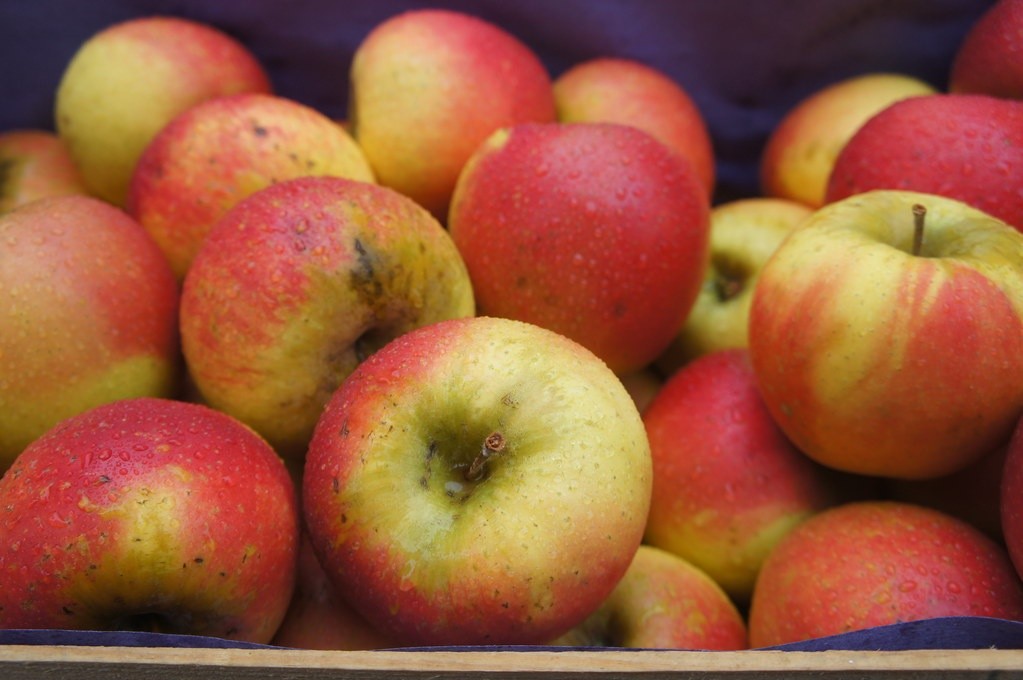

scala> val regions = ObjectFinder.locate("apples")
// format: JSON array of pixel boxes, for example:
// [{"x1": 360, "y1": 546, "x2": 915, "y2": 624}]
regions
[{"x1": 0, "y1": 6, "x2": 1023, "y2": 649}]
